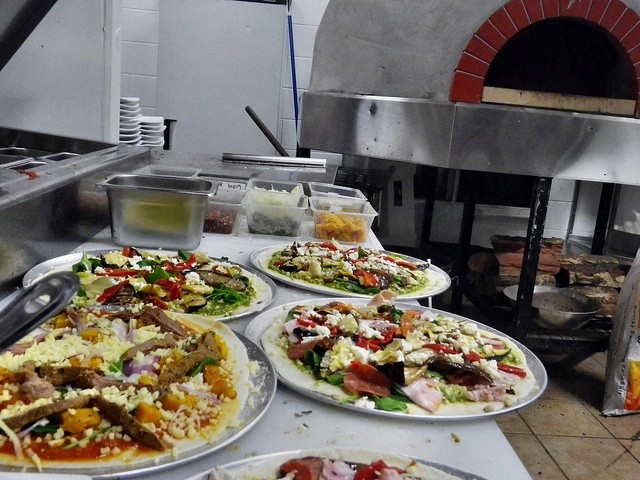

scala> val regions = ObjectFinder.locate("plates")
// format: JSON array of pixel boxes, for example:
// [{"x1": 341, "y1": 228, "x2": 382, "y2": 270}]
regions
[
  {"x1": 241, "y1": 296, "x2": 551, "y2": 424},
  {"x1": 21, "y1": 244, "x2": 277, "y2": 324},
  {"x1": 118, "y1": 95, "x2": 167, "y2": 151},
  {"x1": 186, "y1": 447, "x2": 494, "y2": 480},
  {"x1": 0, "y1": 302, "x2": 277, "y2": 479}
]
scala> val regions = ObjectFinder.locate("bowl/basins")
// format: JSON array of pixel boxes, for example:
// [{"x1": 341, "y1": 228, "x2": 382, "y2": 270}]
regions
[{"x1": 502, "y1": 283, "x2": 602, "y2": 331}]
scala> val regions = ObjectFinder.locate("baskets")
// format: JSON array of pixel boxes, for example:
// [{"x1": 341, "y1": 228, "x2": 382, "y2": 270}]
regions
[{"x1": 491, "y1": 235, "x2": 564, "y2": 254}]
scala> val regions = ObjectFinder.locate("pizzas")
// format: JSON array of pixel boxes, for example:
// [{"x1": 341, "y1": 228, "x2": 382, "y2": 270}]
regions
[
  {"x1": 208, "y1": 448, "x2": 464, "y2": 480},
  {"x1": 33, "y1": 247, "x2": 272, "y2": 316},
  {"x1": 260, "y1": 300, "x2": 534, "y2": 417},
  {"x1": 0, "y1": 305, "x2": 249, "y2": 468}
]
